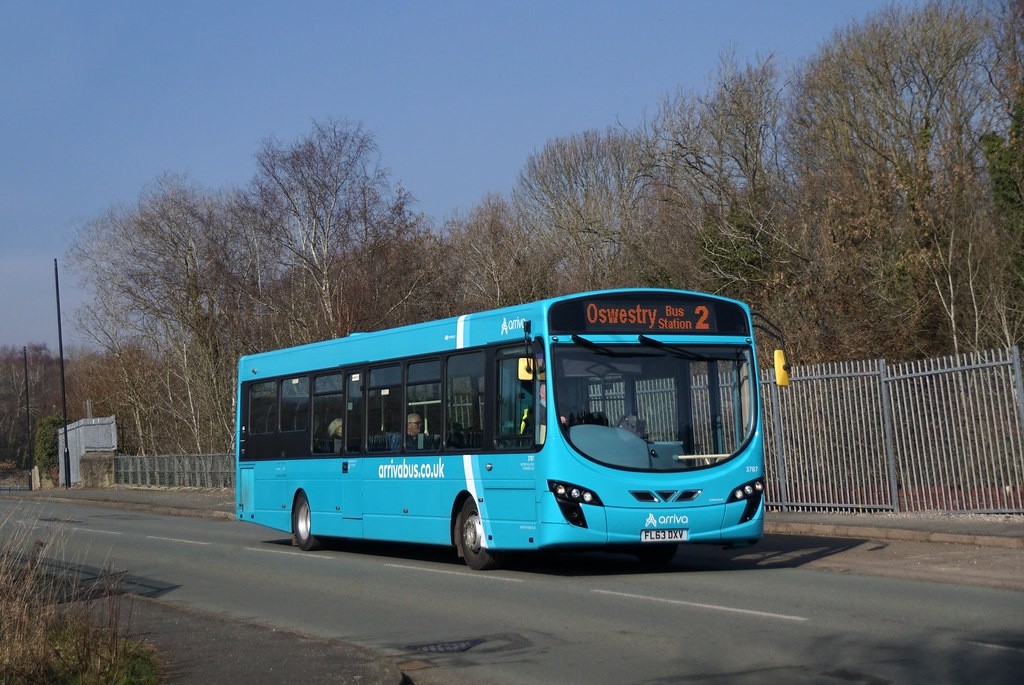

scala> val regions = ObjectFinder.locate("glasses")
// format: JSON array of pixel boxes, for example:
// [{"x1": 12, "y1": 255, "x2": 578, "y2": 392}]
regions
[{"x1": 410, "y1": 421, "x2": 423, "y2": 425}]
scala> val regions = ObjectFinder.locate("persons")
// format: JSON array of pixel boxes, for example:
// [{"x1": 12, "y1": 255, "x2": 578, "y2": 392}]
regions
[
  {"x1": 326, "y1": 418, "x2": 359, "y2": 453},
  {"x1": 527, "y1": 383, "x2": 574, "y2": 434},
  {"x1": 390, "y1": 412, "x2": 435, "y2": 451}
]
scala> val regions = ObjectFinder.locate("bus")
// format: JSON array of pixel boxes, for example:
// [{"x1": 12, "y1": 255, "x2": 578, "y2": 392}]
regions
[{"x1": 234, "y1": 284, "x2": 791, "y2": 578}]
